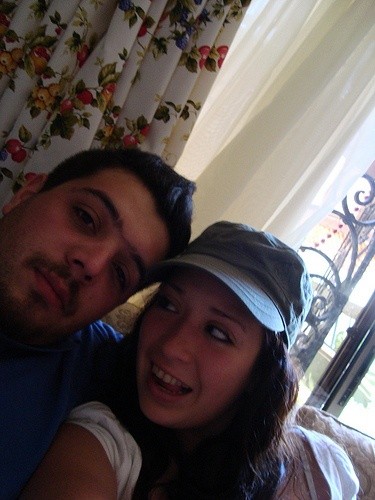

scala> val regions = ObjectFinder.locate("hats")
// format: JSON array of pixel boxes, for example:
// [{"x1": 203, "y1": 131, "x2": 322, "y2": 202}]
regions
[{"x1": 158, "y1": 220, "x2": 314, "y2": 354}]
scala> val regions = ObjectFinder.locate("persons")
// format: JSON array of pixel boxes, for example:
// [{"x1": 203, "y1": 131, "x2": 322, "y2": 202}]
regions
[
  {"x1": 0, "y1": 148, "x2": 194, "y2": 500},
  {"x1": 19, "y1": 222, "x2": 365, "y2": 500}
]
